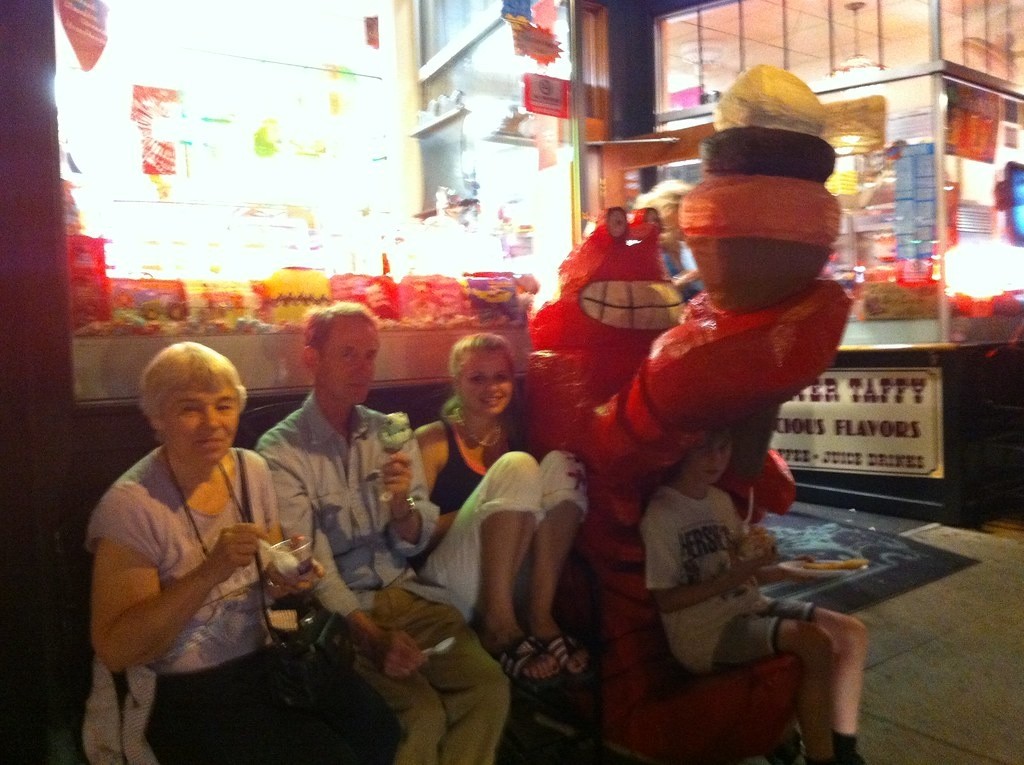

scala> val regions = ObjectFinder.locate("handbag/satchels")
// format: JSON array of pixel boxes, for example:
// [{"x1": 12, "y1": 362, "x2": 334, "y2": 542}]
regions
[{"x1": 275, "y1": 609, "x2": 354, "y2": 705}]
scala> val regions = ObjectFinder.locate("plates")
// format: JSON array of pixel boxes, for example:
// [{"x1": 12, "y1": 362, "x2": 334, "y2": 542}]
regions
[{"x1": 779, "y1": 559, "x2": 869, "y2": 578}]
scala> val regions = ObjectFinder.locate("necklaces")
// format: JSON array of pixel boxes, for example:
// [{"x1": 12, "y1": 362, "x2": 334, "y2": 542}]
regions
[
  {"x1": 456, "y1": 408, "x2": 503, "y2": 446},
  {"x1": 163, "y1": 445, "x2": 248, "y2": 558}
]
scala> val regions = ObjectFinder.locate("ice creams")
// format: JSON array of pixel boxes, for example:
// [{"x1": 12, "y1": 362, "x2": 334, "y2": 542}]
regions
[{"x1": 378, "y1": 411, "x2": 414, "y2": 454}]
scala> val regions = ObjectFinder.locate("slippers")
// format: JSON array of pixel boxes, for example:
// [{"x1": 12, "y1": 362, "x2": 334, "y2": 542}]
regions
[
  {"x1": 490, "y1": 635, "x2": 561, "y2": 688},
  {"x1": 533, "y1": 634, "x2": 594, "y2": 677}
]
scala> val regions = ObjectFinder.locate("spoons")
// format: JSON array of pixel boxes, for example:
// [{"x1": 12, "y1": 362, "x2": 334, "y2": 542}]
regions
[
  {"x1": 421, "y1": 637, "x2": 454, "y2": 655},
  {"x1": 258, "y1": 537, "x2": 299, "y2": 575}
]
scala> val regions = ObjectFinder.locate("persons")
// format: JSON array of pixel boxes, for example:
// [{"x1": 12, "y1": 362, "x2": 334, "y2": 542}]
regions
[
  {"x1": 414, "y1": 333, "x2": 599, "y2": 691},
  {"x1": 641, "y1": 424, "x2": 867, "y2": 765},
  {"x1": 256, "y1": 303, "x2": 510, "y2": 764},
  {"x1": 87, "y1": 341, "x2": 400, "y2": 764}
]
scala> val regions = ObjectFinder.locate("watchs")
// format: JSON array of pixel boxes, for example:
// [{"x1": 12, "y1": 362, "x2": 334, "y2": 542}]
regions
[{"x1": 390, "y1": 497, "x2": 415, "y2": 523}]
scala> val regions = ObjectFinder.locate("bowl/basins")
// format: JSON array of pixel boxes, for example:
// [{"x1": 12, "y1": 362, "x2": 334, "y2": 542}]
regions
[{"x1": 269, "y1": 536, "x2": 315, "y2": 577}]
